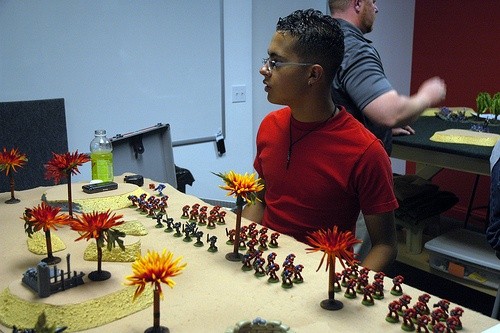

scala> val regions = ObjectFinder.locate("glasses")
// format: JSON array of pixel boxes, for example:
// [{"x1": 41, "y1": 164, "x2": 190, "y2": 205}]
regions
[{"x1": 262, "y1": 57, "x2": 312, "y2": 71}]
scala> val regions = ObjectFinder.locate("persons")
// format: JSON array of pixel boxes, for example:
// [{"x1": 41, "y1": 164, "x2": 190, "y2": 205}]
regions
[
  {"x1": 328, "y1": 0, "x2": 447, "y2": 263},
  {"x1": 231, "y1": 7, "x2": 400, "y2": 276}
]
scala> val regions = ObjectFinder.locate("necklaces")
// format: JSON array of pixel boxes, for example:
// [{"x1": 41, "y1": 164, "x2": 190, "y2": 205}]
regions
[{"x1": 284, "y1": 103, "x2": 338, "y2": 171}]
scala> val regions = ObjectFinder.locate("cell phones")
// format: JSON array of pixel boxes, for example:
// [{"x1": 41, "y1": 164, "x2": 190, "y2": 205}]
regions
[{"x1": 82, "y1": 181, "x2": 118, "y2": 194}]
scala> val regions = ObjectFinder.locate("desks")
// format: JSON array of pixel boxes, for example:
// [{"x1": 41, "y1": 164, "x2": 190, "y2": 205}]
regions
[
  {"x1": 368, "y1": 105, "x2": 500, "y2": 299},
  {"x1": 0, "y1": 172, "x2": 500, "y2": 333}
]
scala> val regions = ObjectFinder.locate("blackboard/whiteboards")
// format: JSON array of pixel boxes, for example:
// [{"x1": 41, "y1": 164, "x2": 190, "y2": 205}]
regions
[{"x1": 0, "y1": 0, "x2": 225, "y2": 155}]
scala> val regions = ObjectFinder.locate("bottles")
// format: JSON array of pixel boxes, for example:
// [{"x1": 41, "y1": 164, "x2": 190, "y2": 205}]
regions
[{"x1": 90, "y1": 130, "x2": 113, "y2": 183}]
